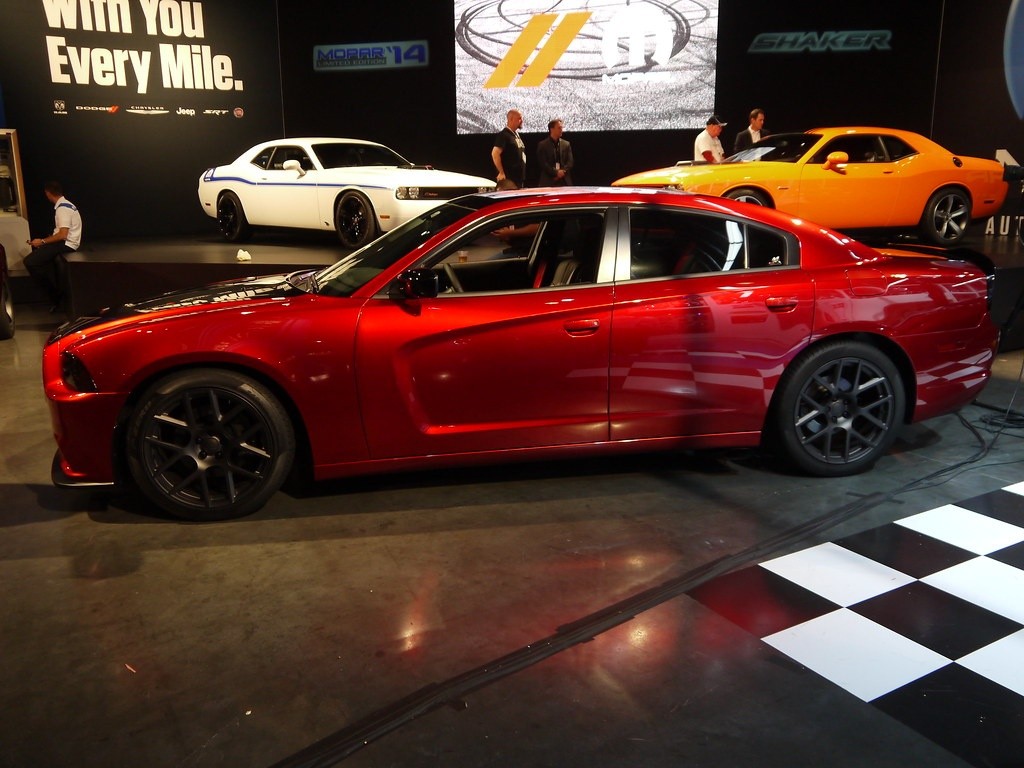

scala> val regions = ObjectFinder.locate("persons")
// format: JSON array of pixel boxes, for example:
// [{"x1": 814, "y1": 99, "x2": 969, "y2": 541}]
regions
[
  {"x1": 694, "y1": 116, "x2": 728, "y2": 164},
  {"x1": 483, "y1": 180, "x2": 540, "y2": 261},
  {"x1": 733, "y1": 109, "x2": 774, "y2": 153},
  {"x1": 23, "y1": 182, "x2": 83, "y2": 313},
  {"x1": 532, "y1": 120, "x2": 574, "y2": 187},
  {"x1": 491, "y1": 111, "x2": 526, "y2": 189}
]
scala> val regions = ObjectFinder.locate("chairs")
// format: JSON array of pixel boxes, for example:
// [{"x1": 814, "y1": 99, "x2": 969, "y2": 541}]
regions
[
  {"x1": 531, "y1": 221, "x2": 579, "y2": 290},
  {"x1": 547, "y1": 226, "x2": 602, "y2": 287}
]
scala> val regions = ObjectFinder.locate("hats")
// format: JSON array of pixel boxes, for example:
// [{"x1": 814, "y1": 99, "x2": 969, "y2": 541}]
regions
[{"x1": 706, "y1": 116, "x2": 728, "y2": 127}]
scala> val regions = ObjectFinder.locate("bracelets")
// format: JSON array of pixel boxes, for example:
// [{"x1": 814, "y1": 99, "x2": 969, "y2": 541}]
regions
[{"x1": 41, "y1": 238, "x2": 47, "y2": 245}]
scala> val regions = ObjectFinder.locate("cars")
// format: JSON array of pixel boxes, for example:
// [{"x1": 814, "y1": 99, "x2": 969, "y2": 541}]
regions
[
  {"x1": 196, "y1": 136, "x2": 498, "y2": 249},
  {"x1": 612, "y1": 127, "x2": 1006, "y2": 248},
  {"x1": 40, "y1": 182, "x2": 996, "y2": 523}
]
